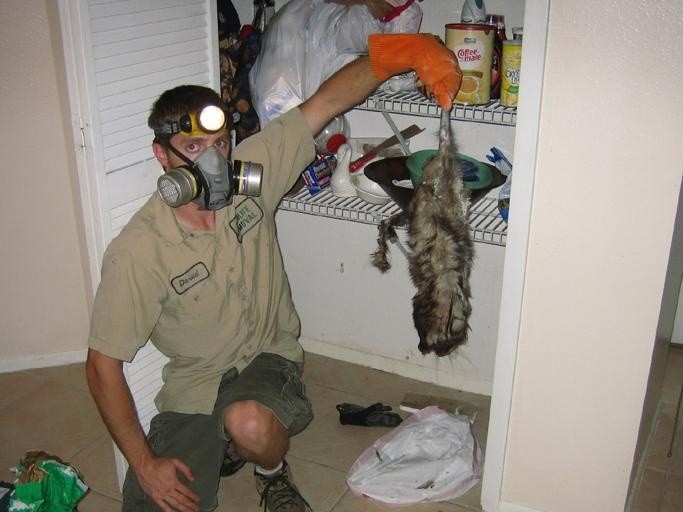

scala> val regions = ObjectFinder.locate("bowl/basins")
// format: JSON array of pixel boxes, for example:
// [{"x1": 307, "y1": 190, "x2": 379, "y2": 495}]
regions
[{"x1": 349, "y1": 173, "x2": 393, "y2": 206}]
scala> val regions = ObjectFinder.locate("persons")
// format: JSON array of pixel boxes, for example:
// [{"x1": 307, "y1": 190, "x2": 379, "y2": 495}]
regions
[{"x1": 83, "y1": 31, "x2": 460, "y2": 511}]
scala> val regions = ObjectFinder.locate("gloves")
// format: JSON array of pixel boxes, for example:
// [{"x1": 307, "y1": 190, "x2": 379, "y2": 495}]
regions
[{"x1": 369, "y1": 32, "x2": 460, "y2": 110}]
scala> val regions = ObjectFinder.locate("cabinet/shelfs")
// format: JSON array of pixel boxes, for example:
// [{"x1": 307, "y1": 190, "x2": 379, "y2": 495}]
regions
[{"x1": 214, "y1": 0, "x2": 532, "y2": 398}]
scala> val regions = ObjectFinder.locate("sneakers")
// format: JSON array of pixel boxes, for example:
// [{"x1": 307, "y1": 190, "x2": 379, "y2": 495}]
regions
[
  {"x1": 254, "y1": 464, "x2": 310, "y2": 511},
  {"x1": 220, "y1": 441, "x2": 246, "y2": 476}
]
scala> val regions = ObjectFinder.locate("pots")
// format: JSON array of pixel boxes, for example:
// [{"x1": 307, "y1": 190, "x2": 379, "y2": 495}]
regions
[{"x1": 363, "y1": 155, "x2": 508, "y2": 212}]
